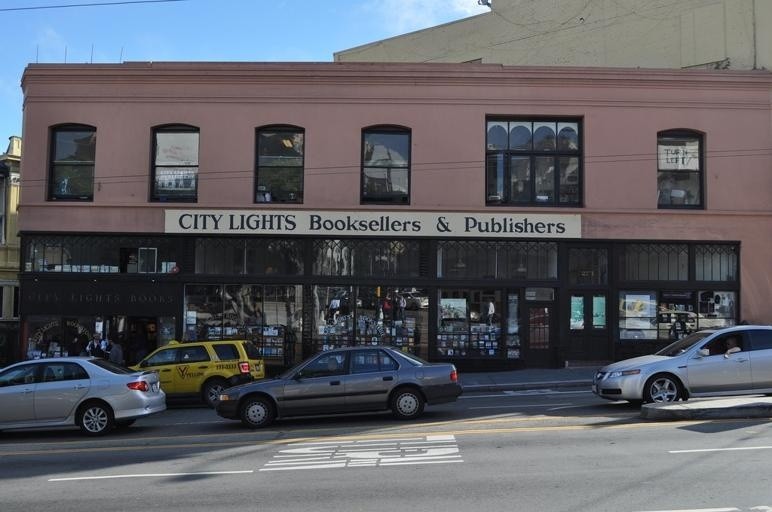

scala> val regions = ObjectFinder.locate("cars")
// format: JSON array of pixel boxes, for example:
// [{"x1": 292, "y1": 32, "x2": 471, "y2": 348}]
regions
[
  {"x1": 620, "y1": 298, "x2": 729, "y2": 345},
  {"x1": 214, "y1": 342, "x2": 466, "y2": 432},
  {"x1": 398, "y1": 291, "x2": 429, "y2": 310},
  {"x1": 1, "y1": 353, "x2": 170, "y2": 438},
  {"x1": 523, "y1": 314, "x2": 550, "y2": 345}
]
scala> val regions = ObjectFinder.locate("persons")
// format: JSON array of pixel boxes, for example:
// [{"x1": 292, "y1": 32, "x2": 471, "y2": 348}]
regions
[
  {"x1": 327, "y1": 357, "x2": 338, "y2": 371},
  {"x1": 66, "y1": 333, "x2": 84, "y2": 357},
  {"x1": 105, "y1": 335, "x2": 123, "y2": 365},
  {"x1": 722, "y1": 334, "x2": 742, "y2": 359},
  {"x1": 86, "y1": 332, "x2": 107, "y2": 357}
]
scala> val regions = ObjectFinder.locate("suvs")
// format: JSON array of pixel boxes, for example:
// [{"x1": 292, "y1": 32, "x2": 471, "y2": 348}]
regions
[
  {"x1": 592, "y1": 323, "x2": 771, "y2": 405},
  {"x1": 124, "y1": 335, "x2": 269, "y2": 411}
]
